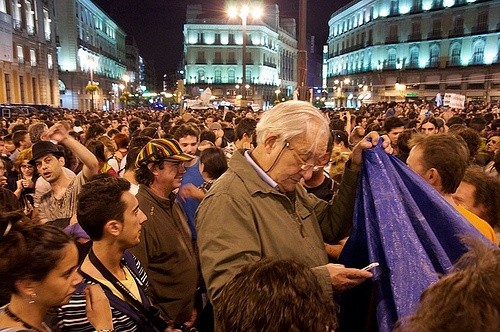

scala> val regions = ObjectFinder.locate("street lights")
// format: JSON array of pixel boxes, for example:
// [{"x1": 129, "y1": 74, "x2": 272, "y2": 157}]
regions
[
  {"x1": 87, "y1": 56, "x2": 94, "y2": 110},
  {"x1": 225, "y1": 3, "x2": 264, "y2": 100},
  {"x1": 334, "y1": 79, "x2": 350, "y2": 107},
  {"x1": 122, "y1": 74, "x2": 130, "y2": 105}
]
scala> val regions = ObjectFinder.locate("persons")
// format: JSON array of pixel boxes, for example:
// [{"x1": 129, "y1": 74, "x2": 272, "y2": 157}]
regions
[
  {"x1": 0, "y1": 97, "x2": 498, "y2": 332},
  {"x1": 195, "y1": 99, "x2": 373, "y2": 332}
]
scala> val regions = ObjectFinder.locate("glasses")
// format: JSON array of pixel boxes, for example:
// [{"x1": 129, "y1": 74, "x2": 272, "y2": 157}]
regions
[{"x1": 285, "y1": 139, "x2": 323, "y2": 172}]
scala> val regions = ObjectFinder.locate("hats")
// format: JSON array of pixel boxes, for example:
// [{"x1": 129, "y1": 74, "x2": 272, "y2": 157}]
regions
[
  {"x1": 135, "y1": 139, "x2": 196, "y2": 168},
  {"x1": 28, "y1": 141, "x2": 63, "y2": 166}
]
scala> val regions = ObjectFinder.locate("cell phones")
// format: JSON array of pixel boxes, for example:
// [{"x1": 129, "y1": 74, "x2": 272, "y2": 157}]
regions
[{"x1": 362, "y1": 263, "x2": 379, "y2": 271}]
